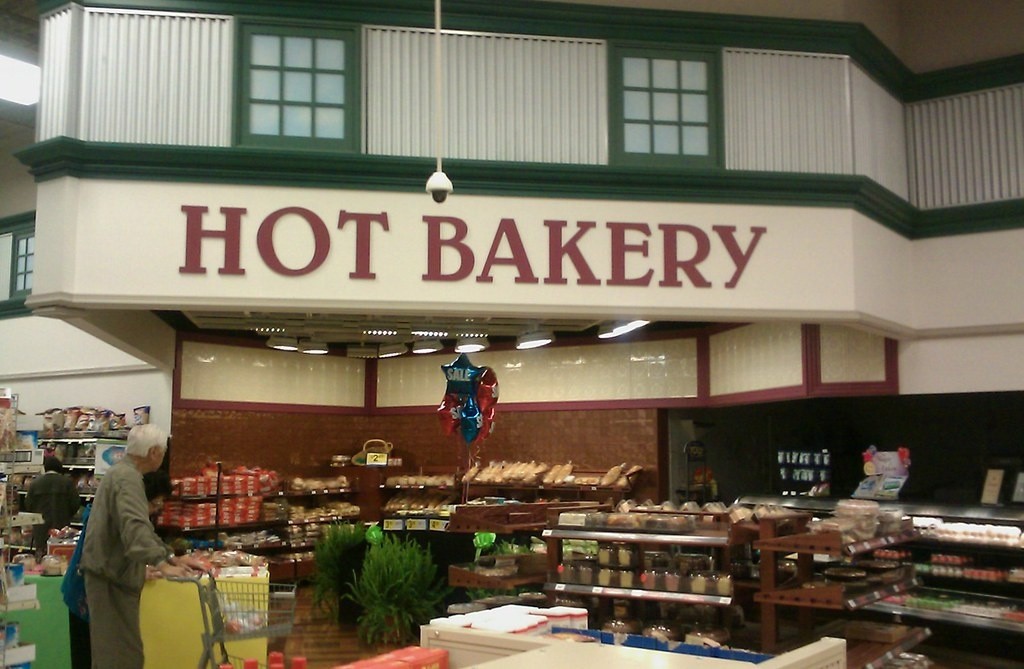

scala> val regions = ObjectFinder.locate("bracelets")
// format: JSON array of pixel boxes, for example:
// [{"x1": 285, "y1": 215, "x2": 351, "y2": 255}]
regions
[{"x1": 168, "y1": 552, "x2": 175, "y2": 560}]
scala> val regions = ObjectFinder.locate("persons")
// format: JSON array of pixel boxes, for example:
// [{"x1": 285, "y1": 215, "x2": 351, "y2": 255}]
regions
[
  {"x1": 25, "y1": 455, "x2": 79, "y2": 561},
  {"x1": 64, "y1": 471, "x2": 79, "y2": 490},
  {"x1": 80, "y1": 425, "x2": 196, "y2": 669},
  {"x1": 60, "y1": 467, "x2": 174, "y2": 669}
]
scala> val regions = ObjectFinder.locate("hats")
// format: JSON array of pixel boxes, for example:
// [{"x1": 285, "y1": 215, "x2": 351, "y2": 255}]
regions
[{"x1": 44, "y1": 456, "x2": 62, "y2": 474}]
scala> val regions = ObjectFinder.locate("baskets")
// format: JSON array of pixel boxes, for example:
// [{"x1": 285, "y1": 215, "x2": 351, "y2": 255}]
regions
[{"x1": 350, "y1": 439, "x2": 393, "y2": 467}]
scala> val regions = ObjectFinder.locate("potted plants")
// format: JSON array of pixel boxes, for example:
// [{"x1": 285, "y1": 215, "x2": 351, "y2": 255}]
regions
[{"x1": 339, "y1": 532, "x2": 453, "y2": 648}]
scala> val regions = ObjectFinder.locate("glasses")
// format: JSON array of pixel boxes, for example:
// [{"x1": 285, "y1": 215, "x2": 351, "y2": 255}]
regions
[{"x1": 151, "y1": 496, "x2": 164, "y2": 510}]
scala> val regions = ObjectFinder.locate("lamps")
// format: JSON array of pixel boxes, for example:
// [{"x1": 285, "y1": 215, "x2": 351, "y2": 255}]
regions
[
  {"x1": 263, "y1": 317, "x2": 652, "y2": 359},
  {"x1": 0, "y1": 39, "x2": 39, "y2": 106}
]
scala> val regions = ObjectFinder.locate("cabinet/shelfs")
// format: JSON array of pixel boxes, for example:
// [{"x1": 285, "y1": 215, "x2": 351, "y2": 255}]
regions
[
  {"x1": 160, "y1": 457, "x2": 1024, "y2": 669},
  {"x1": 0, "y1": 438, "x2": 127, "y2": 553}
]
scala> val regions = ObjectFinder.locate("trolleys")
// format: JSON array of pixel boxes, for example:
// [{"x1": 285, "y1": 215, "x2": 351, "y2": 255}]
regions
[{"x1": 165, "y1": 567, "x2": 296, "y2": 669}]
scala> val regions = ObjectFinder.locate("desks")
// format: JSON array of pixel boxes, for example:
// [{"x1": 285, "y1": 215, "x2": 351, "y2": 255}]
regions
[{"x1": 0, "y1": 569, "x2": 268, "y2": 669}]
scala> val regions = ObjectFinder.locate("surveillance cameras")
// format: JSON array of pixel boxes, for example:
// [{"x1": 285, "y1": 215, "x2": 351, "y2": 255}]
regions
[{"x1": 424, "y1": 172, "x2": 453, "y2": 203}]
